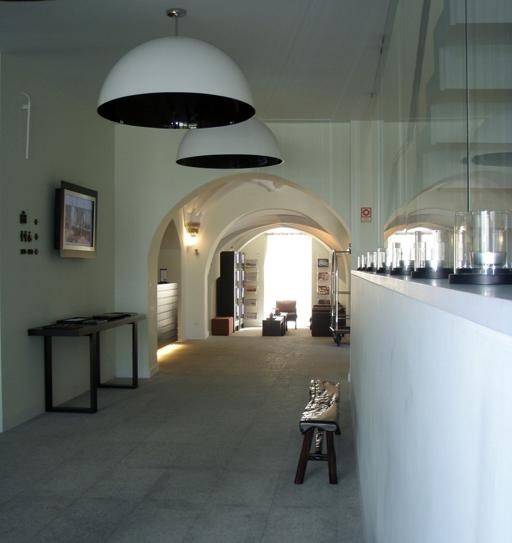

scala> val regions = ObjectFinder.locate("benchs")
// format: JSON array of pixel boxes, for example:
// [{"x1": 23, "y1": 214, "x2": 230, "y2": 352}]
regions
[{"x1": 290, "y1": 378, "x2": 342, "y2": 484}]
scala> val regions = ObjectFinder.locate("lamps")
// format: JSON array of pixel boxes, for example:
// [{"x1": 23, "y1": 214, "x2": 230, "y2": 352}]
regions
[
  {"x1": 96, "y1": 5, "x2": 258, "y2": 129},
  {"x1": 175, "y1": 114, "x2": 284, "y2": 170}
]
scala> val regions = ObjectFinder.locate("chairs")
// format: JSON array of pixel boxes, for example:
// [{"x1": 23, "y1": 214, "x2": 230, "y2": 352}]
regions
[{"x1": 273, "y1": 298, "x2": 297, "y2": 330}]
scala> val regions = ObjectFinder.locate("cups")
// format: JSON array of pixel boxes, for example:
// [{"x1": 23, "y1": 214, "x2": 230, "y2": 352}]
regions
[
  {"x1": 266, "y1": 307, "x2": 280, "y2": 321},
  {"x1": 412, "y1": 231, "x2": 450, "y2": 278},
  {"x1": 357, "y1": 247, "x2": 394, "y2": 273},
  {"x1": 391, "y1": 241, "x2": 416, "y2": 275},
  {"x1": 450, "y1": 209, "x2": 512, "y2": 284}
]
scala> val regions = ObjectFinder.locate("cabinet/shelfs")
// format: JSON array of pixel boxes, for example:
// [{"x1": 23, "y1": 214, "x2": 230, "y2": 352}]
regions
[{"x1": 216, "y1": 248, "x2": 245, "y2": 330}]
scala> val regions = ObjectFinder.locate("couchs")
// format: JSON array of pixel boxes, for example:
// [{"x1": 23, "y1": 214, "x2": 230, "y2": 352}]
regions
[{"x1": 309, "y1": 297, "x2": 347, "y2": 337}]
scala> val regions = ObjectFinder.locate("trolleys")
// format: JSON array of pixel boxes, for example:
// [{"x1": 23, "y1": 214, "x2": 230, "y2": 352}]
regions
[{"x1": 325, "y1": 244, "x2": 352, "y2": 348}]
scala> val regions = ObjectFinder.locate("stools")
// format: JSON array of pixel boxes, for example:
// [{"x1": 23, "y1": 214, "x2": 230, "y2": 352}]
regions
[{"x1": 210, "y1": 316, "x2": 234, "y2": 336}]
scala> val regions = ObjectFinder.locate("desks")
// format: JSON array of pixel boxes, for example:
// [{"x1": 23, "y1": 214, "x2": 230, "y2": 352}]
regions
[{"x1": 29, "y1": 311, "x2": 147, "y2": 413}]
